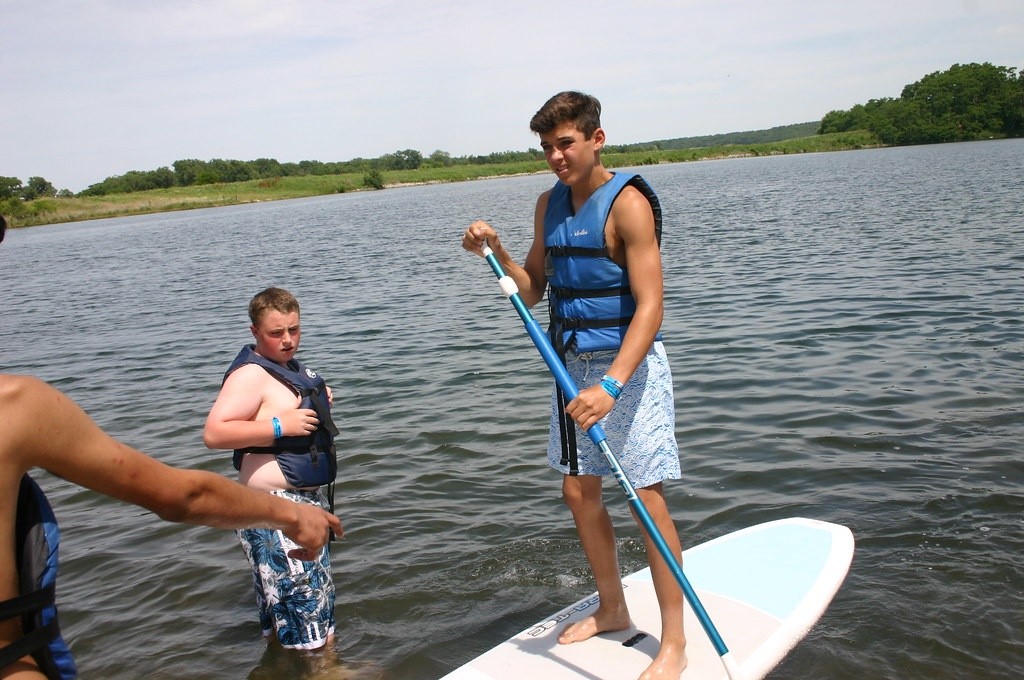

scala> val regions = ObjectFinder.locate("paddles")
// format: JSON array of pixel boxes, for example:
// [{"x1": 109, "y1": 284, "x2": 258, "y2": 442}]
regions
[{"x1": 480, "y1": 239, "x2": 745, "y2": 680}]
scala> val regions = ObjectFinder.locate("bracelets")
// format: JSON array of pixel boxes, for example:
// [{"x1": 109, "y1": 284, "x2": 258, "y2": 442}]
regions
[
  {"x1": 272, "y1": 417, "x2": 282, "y2": 439},
  {"x1": 598, "y1": 374, "x2": 624, "y2": 401}
]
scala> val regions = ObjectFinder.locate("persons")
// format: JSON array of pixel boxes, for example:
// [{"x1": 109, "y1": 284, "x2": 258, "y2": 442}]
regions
[
  {"x1": 203, "y1": 288, "x2": 340, "y2": 675},
  {"x1": 463, "y1": 92, "x2": 688, "y2": 679},
  {"x1": 1, "y1": 218, "x2": 344, "y2": 680}
]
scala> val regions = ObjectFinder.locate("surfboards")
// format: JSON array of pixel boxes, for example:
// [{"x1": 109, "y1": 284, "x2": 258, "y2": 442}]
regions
[{"x1": 435, "y1": 516, "x2": 857, "y2": 680}]
